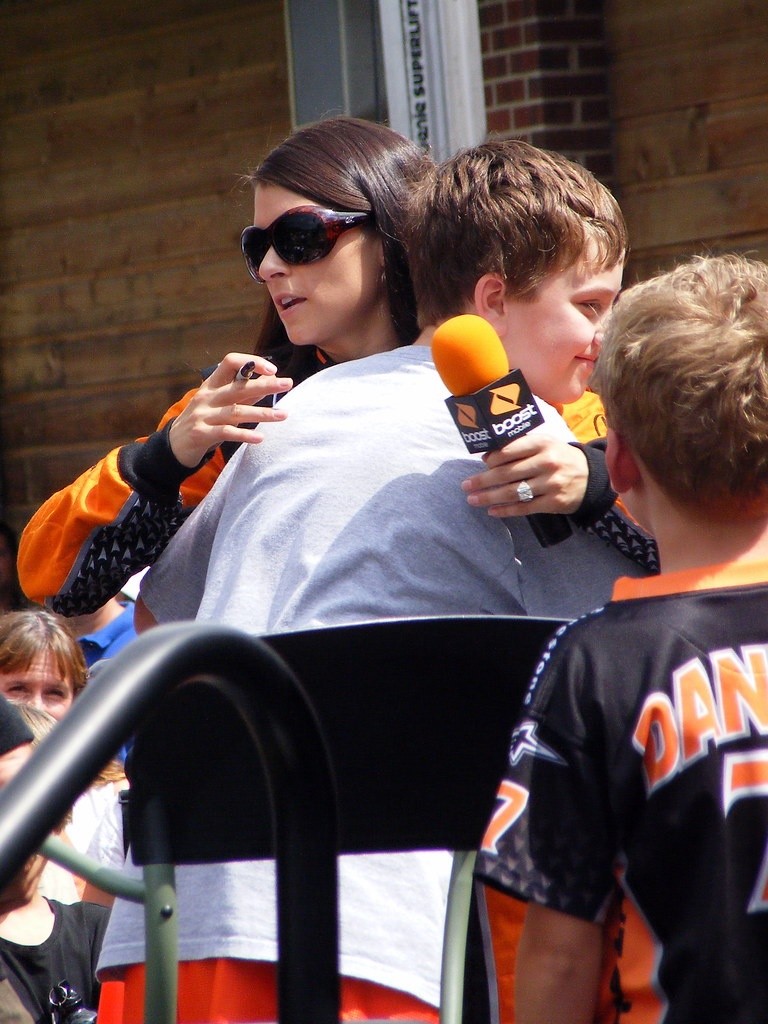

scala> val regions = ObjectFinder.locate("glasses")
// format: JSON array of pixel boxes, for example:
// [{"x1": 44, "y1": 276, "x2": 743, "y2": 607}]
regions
[{"x1": 240, "y1": 205, "x2": 380, "y2": 284}]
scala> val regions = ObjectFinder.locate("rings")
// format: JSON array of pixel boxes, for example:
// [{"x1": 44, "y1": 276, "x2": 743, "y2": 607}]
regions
[{"x1": 516, "y1": 480, "x2": 533, "y2": 503}]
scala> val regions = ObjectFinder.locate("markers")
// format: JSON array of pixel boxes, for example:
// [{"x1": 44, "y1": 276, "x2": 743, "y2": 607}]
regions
[{"x1": 235, "y1": 360, "x2": 256, "y2": 382}]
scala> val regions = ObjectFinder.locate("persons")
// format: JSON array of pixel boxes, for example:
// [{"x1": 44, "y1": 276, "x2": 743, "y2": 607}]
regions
[
  {"x1": 472, "y1": 252, "x2": 768, "y2": 1024},
  {"x1": 0, "y1": 591, "x2": 143, "y2": 1024},
  {"x1": 18, "y1": 116, "x2": 655, "y2": 1024},
  {"x1": 91, "y1": 140, "x2": 658, "y2": 1024}
]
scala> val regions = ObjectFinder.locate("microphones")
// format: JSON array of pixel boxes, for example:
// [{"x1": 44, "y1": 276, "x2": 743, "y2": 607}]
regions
[{"x1": 431, "y1": 313, "x2": 574, "y2": 549}]
